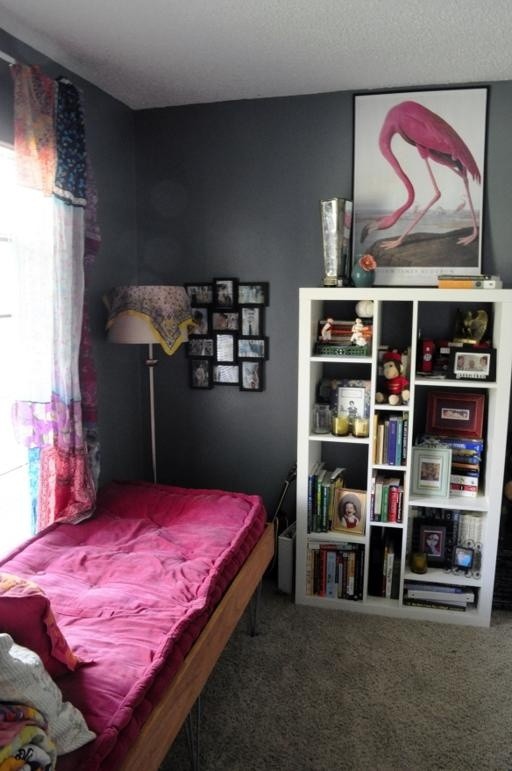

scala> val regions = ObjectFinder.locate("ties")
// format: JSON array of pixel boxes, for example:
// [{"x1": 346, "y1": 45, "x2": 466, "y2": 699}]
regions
[{"x1": 248, "y1": 324, "x2": 252, "y2": 334}]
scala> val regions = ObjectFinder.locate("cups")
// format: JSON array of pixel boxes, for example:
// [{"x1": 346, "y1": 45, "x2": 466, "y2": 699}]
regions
[
  {"x1": 411, "y1": 551, "x2": 428, "y2": 574},
  {"x1": 330, "y1": 410, "x2": 350, "y2": 436},
  {"x1": 352, "y1": 414, "x2": 369, "y2": 437}
]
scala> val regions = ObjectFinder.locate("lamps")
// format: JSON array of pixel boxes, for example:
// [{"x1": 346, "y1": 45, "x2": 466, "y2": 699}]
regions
[{"x1": 99, "y1": 281, "x2": 196, "y2": 488}]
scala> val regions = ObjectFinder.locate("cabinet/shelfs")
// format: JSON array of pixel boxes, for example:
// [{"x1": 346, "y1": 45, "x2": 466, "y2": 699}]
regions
[{"x1": 293, "y1": 281, "x2": 511, "y2": 633}]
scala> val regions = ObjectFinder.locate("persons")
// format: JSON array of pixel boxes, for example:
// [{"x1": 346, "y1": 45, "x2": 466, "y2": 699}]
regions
[
  {"x1": 195, "y1": 363, "x2": 205, "y2": 386},
  {"x1": 348, "y1": 400, "x2": 358, "y2": 425},
  {"x1": 216, "y1": 284, "x2": 231, "y2": 305},
  {"x1": 191, "y1": 310, "x2": 207, "y2": 334},
  {"x1": 457, "y1": 355, "x2": 488, "y2": 372},
  {"x1": 190, "y1": 288, "x2": 198, "y2": 302},
  {"x1": 215, "y1": 314, "x2": 227, "y2": 330}
]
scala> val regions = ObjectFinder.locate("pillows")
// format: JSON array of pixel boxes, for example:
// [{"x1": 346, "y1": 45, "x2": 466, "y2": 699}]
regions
[
  {"x1": 0, "y1": 631, "x2": 101, "y2": 759},
  {"x1": 0, "y1": 571, "x2": 88, "y2": 673}
]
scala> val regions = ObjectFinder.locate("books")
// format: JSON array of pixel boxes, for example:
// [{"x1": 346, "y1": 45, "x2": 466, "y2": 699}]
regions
[
  {"x1": 306, "y1": 412, "x2": 486, "y2": 610},
  {"x1": 318, "y1": 319, "x2": 372, "y2": 348}
]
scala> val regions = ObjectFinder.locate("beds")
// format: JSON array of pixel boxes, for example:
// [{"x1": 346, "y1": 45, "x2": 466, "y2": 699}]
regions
[{"x1": 1, "y1": 478, "x2": 280, "y2": 771}]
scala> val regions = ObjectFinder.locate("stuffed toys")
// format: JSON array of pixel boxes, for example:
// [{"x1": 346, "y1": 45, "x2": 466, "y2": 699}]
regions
[{"x1": 376, "y1": 348, "x2": 410, "y2": 405}]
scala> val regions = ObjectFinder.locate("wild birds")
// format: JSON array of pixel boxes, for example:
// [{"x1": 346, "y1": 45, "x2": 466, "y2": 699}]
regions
[{"x1": 358, "y1": 99, "x2": 484, "y2": 252}]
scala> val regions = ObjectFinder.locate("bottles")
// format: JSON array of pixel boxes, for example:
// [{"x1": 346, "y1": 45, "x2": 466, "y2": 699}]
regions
[
  {"x1": 337, "y1": 274, "x2": 348, "y2": 287},
  {"x1": 421, "y1": 337, "x2": 436, "y2": 372},
  {"x1": 312, "y1": 403, "x2": 330, "y2": 434}
]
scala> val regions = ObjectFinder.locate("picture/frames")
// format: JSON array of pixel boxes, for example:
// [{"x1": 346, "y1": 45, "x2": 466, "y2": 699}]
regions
[
  {"x1": 184, "y1": 273, "x2": 273, "y2": 394},
  {"x1": 350, "y1": 82, "x2": 495, "y2": 284}
]
